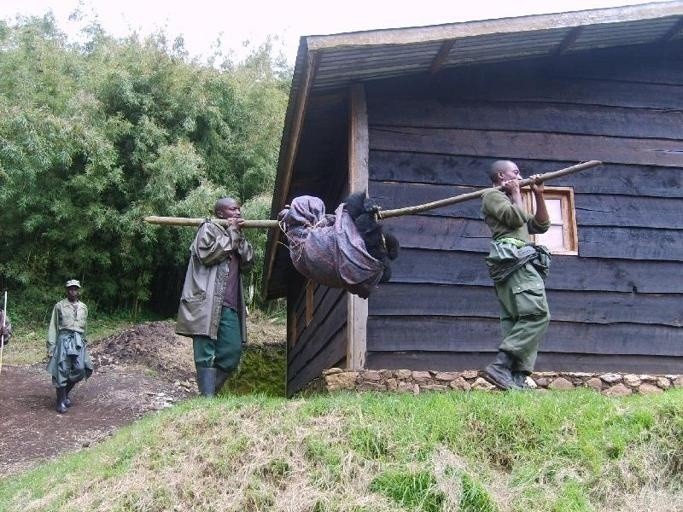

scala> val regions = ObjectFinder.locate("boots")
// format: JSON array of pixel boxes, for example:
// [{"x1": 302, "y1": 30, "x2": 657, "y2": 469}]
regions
[
  {"x1": 195, "y1": 365, "x2": 232, "y2": 399},
  {"x1": 56, "y1": 386, "x2": 72, "y2": 413},
  {"x1": 513, "y1": 371, "x2": 540, "y2": 389},
  {"x1": 478, "y1": 350, "x2": 518, "y2": 390}
]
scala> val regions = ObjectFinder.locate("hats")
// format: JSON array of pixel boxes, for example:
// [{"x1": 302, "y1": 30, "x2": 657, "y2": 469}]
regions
[{"x1": 65, "y1": 279, "x2": 82, "y2": 288}]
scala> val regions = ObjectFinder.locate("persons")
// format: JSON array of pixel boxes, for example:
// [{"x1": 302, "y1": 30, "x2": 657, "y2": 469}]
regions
[
  {"x1": 0, "y1": 308, "x2": 11, "y2": 346},
  {"x1": 175, "y1": 197, "x2": 257, "y2": 397},
  {"x1": 478, "y1": 158, "x2": 554, "y2": 391},
  {"x1": 45, "y1": 279, "x2": 93, "y2": 414}
]
